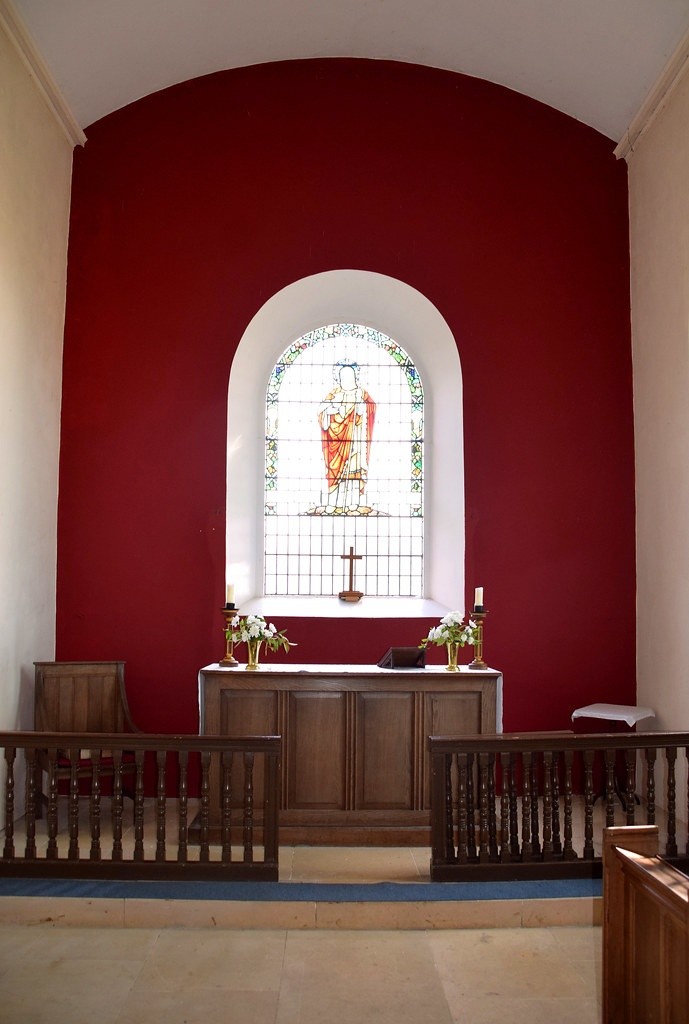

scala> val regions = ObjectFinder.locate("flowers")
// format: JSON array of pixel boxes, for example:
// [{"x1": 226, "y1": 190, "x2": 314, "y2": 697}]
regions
[
  {"x1": 417, "y1": 611, "x2": 483, "y2": 651},
  {"x1": 225, "y1": 608, "x2": 298, "y2": 657}
]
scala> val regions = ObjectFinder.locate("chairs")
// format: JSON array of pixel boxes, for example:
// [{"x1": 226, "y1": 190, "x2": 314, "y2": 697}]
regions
[{"x1": 32, "y1": 659, "x2": 148, "y2": 828}]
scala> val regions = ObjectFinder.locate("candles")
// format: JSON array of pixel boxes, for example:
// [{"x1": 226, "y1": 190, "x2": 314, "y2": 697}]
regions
[
  {"x1": 473, "y1": 586, "x2": 484, "y2": 607},
  {"x1": 225, "y1": 582, "x2": 236, "y2": 603}
]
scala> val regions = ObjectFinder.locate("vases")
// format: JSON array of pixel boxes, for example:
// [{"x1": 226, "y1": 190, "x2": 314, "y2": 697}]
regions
[
  {"x1": 244, "y1": 640, "x2": 262, "y2": 671},
  {"x1": 444, "y1": 641, "x2": 461, "y2": 672}
]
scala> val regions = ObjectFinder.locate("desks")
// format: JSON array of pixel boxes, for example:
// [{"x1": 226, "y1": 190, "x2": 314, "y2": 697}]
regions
[
  {"x1": 182, "y1": 662, "x2": 509, "y2": 850},
  {"x1": 570, "y1": 702, "x2": 656, "y2": 813}
]
